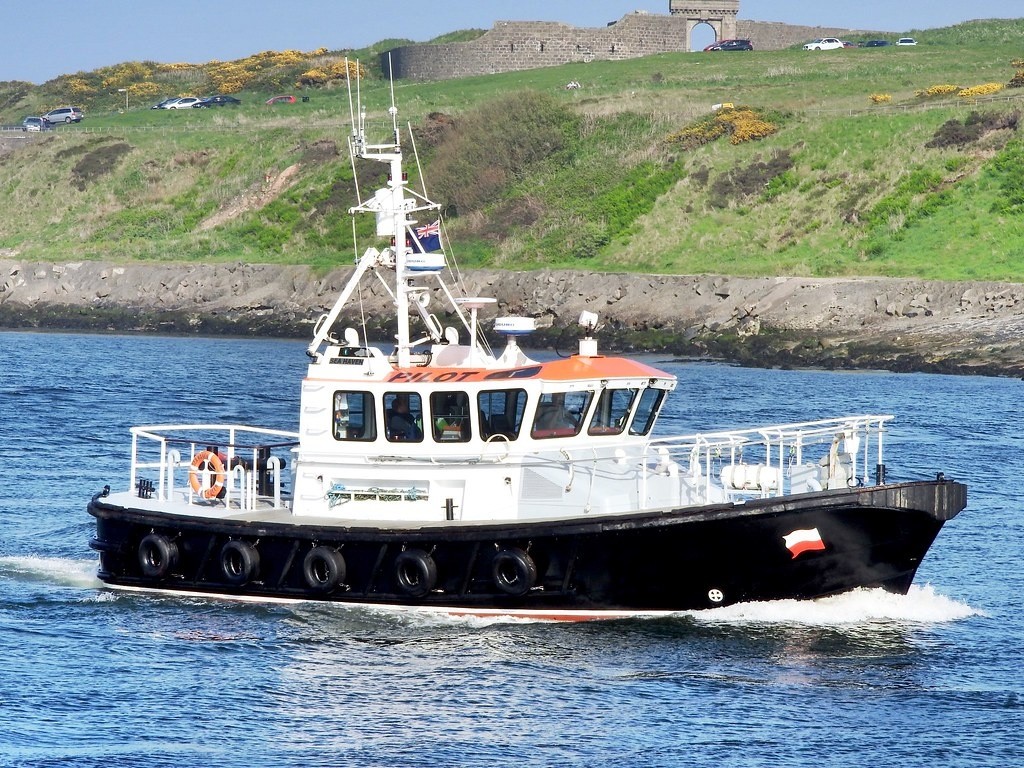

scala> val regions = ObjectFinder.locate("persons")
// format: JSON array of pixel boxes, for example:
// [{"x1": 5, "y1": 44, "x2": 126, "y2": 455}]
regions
[{"x1": 388, "y1": 398, "x2": 422, "y2": 441}]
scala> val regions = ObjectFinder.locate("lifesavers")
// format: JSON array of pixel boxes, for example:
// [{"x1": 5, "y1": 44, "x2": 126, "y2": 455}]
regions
[
  {"x1": 137, "y1": 535, "x2": 180, "y2": 578},
  {"x1": 302, "y1": 546, "x2": 348, "y2": 593},
  {"x1": 395, "y1": 551, "x2": 439, "y2": 597},
  {"x1": 189, "y1": 451, "x2": 227, "y2": 500},
  {"x1": 220, "y1": 540, "x2": 262, "y2": 582},
  {"x1": 490, "y1": 548, "x2": 537, "y2": 598}
]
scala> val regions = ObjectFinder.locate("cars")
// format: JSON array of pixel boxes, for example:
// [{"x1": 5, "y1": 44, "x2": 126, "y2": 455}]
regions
[
  {"x1": 865, "y1": 41, "x2": 890, "y2": 47},
  {"x1": 703, "y1": 39, "x2": 753, "y2": 51},
  {"x1": 151, "y1": 97, "x2": 201, "y2": 110},
  {"x1": 265, "y1": 96, "x2": 296, "y2": 105},
  {"x1": 802, "y1": 38, "x2": 844, "y2": 50},
  {"x1": 842, "y1": 40, "x2": 865, "y2": 48},
  {"x1": 192, "y1": 95, "x2": 241, "y2": 110},
  {"x1": 895, "y1": 38, "x2": 918, "y2": 46}
]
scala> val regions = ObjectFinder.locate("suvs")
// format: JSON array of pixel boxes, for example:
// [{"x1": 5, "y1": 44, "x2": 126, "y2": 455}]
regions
[
  {"x1": 22, "y1": 116, "x2": 50, "y2": 132},
  {"x1": 41, "y1": 107, "x2": 84, "y2": 124}
]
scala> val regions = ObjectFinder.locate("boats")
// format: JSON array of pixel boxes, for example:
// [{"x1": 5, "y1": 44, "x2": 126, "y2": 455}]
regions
[{"x1": 86, "y1": 49, "x2": 969, "y2": 625}]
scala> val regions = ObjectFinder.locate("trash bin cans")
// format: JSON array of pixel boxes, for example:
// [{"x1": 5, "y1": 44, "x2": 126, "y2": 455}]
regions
[{"x1": 302, "y1": 96, "x2": 310, "y2": 102}]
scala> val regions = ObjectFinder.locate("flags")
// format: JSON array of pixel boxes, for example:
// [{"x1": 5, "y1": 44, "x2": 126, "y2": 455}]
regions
[{"x1": 412, "y1": 220, "x2": 444, "y2": 254}]
